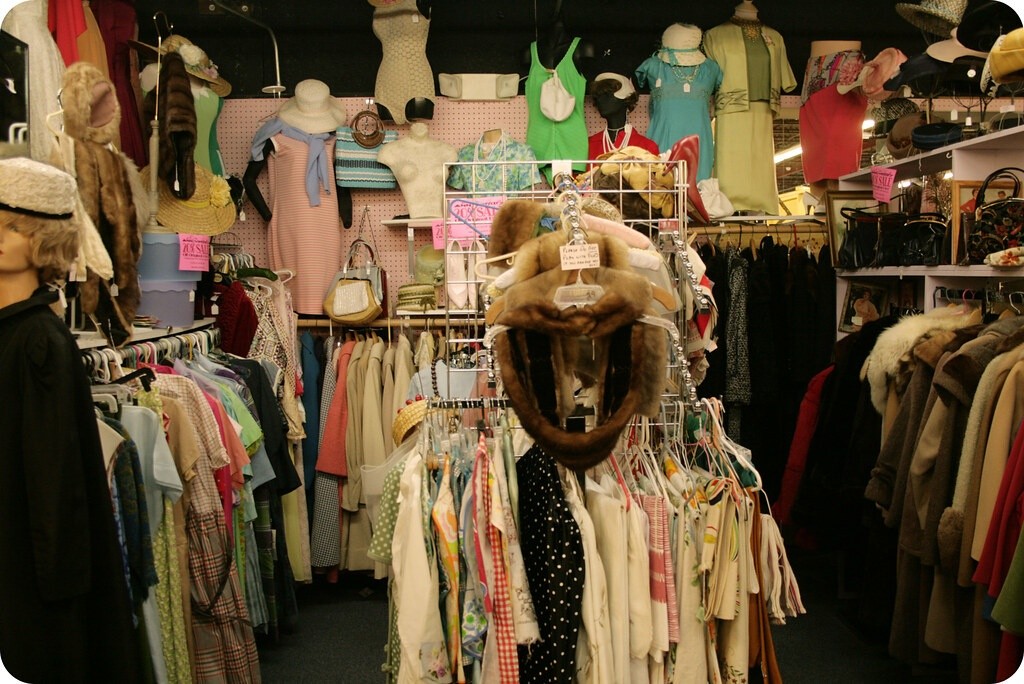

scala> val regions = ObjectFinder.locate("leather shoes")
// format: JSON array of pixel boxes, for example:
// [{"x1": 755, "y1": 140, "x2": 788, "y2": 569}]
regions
[{"x1": 445, "y1": 239, "x2": 488, "y2": 310}]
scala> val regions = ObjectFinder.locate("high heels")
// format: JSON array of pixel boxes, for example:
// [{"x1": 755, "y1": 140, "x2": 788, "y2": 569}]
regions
[{"x1": 662, "y1": 135, "x2": 710, "y2": 225}]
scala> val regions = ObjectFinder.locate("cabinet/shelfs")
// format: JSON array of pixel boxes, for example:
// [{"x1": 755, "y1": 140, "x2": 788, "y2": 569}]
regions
[{"x1": 835, "y1": 123, "x2": 1024, "y2": 345}]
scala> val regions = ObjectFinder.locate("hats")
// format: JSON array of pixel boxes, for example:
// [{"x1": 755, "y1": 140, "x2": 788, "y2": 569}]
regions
[
  {"x1": 60, "y1": 61, "x2": 122, "y2": 144},
  {"x1": 414, "y1": 245, "x2": 444, "y2": 286},
  {"x1": 0, "y1": 157, "x2": 76, "y2": 219},
  {"x1": 540, "y1": 70, "x2": 575, "y2": 122},
  {"x1": 127, "y1": 34, "x2": 232, "y2": 97},
  {"x1": 837, "y1": 0, "x2": 1024, "y2": 162},
  {"x1": 394, "y1": 284, "x2": 437, "y2": 313},
  {"x1": 657, "y1": 22, "x2": 706, "y2": 66},
  {"x1": 139, "y1": 163, "x2": 236, "y2": 237},
  {"x1": 278, "y1": 79, "x2": 347, "y2": 134}
]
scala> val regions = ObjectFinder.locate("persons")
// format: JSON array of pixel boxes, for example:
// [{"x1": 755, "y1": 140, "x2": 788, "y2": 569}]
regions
[
  {"x1": 0, "y1": 156, "x2": 162, "y2": 683},
  {"x1": 48, "y1": 0, "x2": 889, "y2": 323}
]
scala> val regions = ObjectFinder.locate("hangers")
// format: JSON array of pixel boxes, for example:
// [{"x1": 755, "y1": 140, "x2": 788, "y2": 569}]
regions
[
  {"x1": 607, "y1": 396, "x2": 772, "y2": 519},
  {"x1": 690, "y1": 226, "x2": 828, "y2": 265},
  {"x1": 419, "y1": 394, "x2": 509, "y2": 472},
  {"x1": 447, "y1": 182, "x2": 680, "y2": 345},
  {"x1": 81, "y1": 328, "x2": 230, "y2": 413},
  {"x1": 46, "y1": 111, "x2": 122, "y2": 157},
  {"x1": 212, "y1": 253, "x2": 294, "y2": 300},
  {"x1": 326, "y1": 318, "x2": 483, "y2": 351},
  {"x1": 933, "y1": 286, "x2": 1024, "y2": 324}
]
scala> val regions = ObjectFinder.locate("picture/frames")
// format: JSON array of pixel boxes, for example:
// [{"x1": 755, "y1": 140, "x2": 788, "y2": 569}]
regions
[
  {"x1": 951, "y1": 178, "x2": 1024, "y2": 267},
  {"x1": 825, "y1": 188, "x2": 889, "y2": 267},
  {"x1": 838, "y1": 279, "x2": 890, "y2": 334}
]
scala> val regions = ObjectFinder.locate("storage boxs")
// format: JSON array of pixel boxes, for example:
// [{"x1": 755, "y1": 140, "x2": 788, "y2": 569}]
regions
[
  {"x1": 136, "y1": 280, "x2": 199, "y2": 331},
  {"x1": 138, "y1": 232, "x2": 204, "y2": 285}
]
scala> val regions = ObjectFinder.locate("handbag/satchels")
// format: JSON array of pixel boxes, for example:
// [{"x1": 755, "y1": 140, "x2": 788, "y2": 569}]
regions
[
  {"x1": 838, "y1": 167, "x2": 1024, "y2": 272},
  {"x1": 322, "y1": 208, "x2": 393, "y2": 326},
  {"x1": 335, "y1": 111, "x2": 398, "y2": 189}
]
